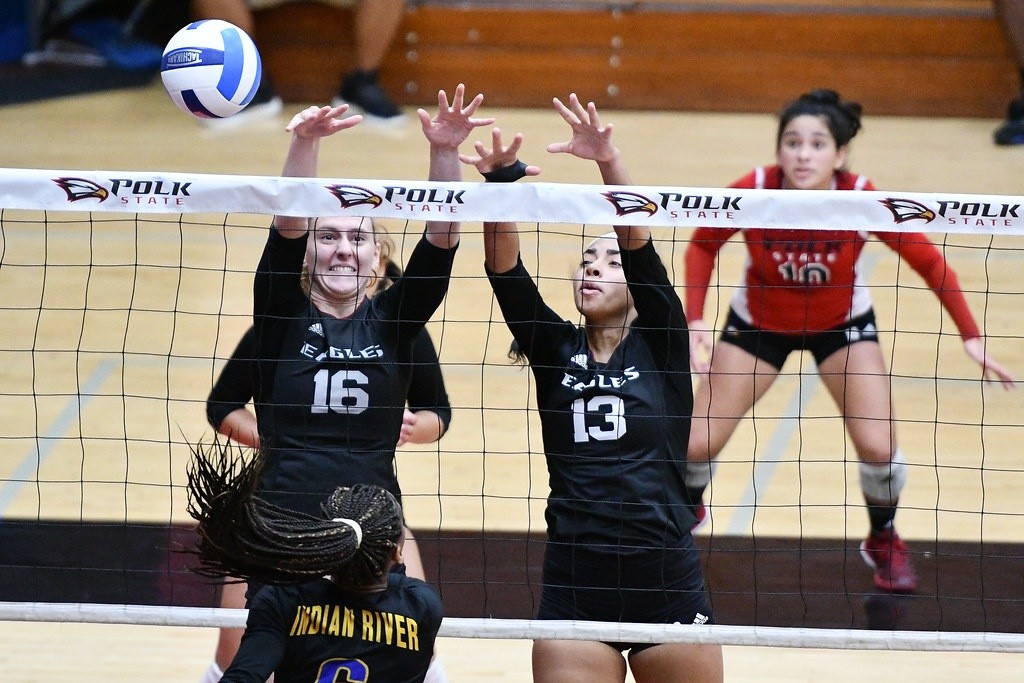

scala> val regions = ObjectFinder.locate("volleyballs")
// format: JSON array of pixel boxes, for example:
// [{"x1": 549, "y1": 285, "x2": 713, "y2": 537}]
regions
[{"x1": 159, "y1": 19, "x2": 264, "y2": 124}]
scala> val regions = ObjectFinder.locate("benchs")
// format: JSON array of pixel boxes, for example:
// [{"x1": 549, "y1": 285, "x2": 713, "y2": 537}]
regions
[{"x1": 249, "y1": 0, "x2": 1024, "y2": 118}]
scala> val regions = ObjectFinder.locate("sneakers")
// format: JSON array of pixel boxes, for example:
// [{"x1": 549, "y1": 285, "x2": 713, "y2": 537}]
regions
[
  {"x1": 689, "y1": 497, "x2": 708, "y2": 535},
  {"x1": 995, "y1": 99, "x2": 1024, "y2": 146},
  {"x1": 333, "y1": 68, "x2": 407, "y2": 129},
  {"x1": 860, "y1": 527, "x2": 918, "y2": 592},
  {"x1": 199, "y1": 72, "x2": 285, "y2": 126}
]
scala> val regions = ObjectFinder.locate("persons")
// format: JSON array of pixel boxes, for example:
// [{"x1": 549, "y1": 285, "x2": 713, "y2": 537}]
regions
[
  {"x1": 207, "y1": 84, "x2": 495, "y2": 683},
  {"x1": 198, "y1": 0, "x2": 407, "y2": 126},
  {"x1": 684, "y1": 90, "x2": 1016, "y2": 588},
  {"x1": 459, "y1": 93, "x2": 724, "y2": 683},
  {"x1": 173, "y1": 426, "x2": 444, "y2": 683},
  {"x1": 992, "y1": 0, "x2": 1024, "y2": 145}
]
